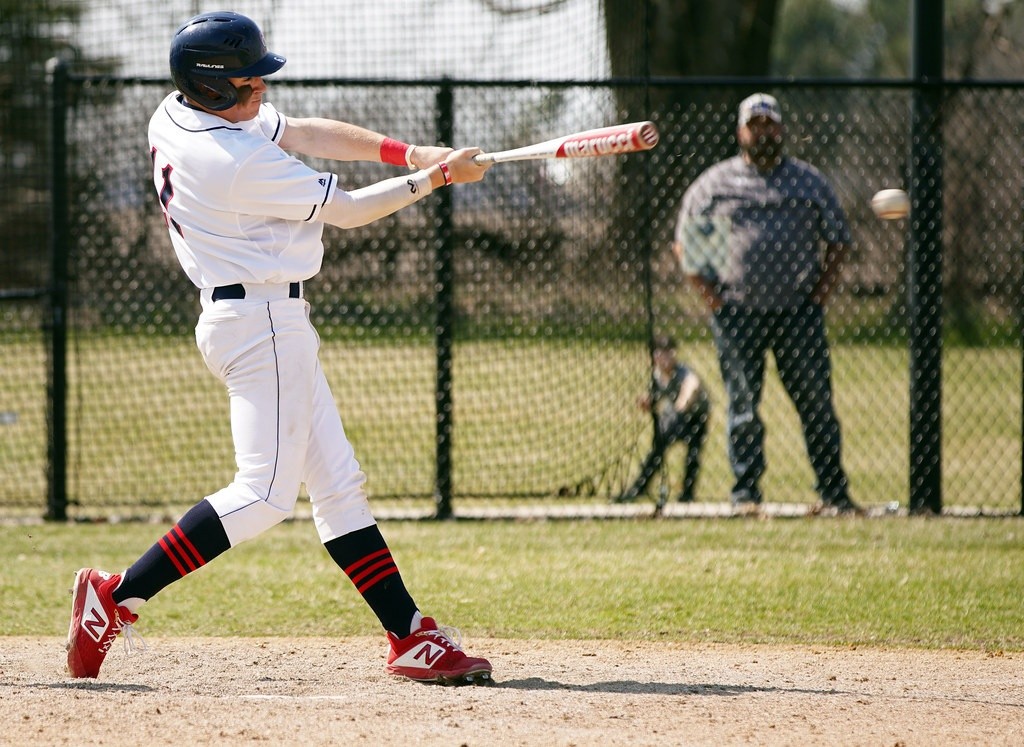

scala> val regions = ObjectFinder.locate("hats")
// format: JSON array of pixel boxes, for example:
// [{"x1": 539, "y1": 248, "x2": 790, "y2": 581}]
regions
[
  {"x1": 647, "y1": 333, "x2": 676, "y2": 347},
  {"x1": 739, "y1": 94, "x2": 782, "y2": 127}
]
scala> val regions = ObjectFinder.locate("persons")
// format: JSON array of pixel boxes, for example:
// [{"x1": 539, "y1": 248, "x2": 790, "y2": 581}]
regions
[
  {"x1": 673, "y1": 89, "x2": 863, "y2": 513},
  {"x1": 613, "y1": 332, "x2": 712, "y2": 506},
  {"x1": 63, "y1": 10, "x2": 496, "y2": 686}
]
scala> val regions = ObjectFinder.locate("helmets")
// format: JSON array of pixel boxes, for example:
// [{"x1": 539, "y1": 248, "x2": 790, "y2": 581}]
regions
[{"x1": 169, "y1": 11, "x2": 287, "y2": 113}]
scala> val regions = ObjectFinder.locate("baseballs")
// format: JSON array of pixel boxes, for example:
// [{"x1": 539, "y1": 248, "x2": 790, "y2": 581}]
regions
[{"x1": 870, "y1": 188, "x2": 911, "y2": 219}]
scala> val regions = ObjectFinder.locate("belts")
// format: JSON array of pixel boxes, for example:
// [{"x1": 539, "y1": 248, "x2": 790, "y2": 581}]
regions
[{"x1": 199, "y1": 282, "x2": 305, "y2": 299}]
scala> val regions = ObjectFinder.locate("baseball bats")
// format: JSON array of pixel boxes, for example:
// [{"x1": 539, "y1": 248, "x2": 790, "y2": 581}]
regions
[{"x1": 472, "y1": 120, "x2": 661, "y2": 167}]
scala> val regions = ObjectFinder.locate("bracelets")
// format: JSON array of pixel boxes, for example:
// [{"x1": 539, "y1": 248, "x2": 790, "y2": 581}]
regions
[
  {"x1": 405, "y1": 144, "x2": 418, "y2": 171},
  {"x1": 437, "y1": 161, "x2": 453, "y2": 186}
]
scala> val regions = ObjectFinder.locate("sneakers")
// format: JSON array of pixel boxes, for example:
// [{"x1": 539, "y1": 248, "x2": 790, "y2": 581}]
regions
[
  {"x1": 382, "y1": 616, "x2": 492, "y2": 684},
  {"x1": 62, "y1": 568, "x2": 146, "y2": 678}
]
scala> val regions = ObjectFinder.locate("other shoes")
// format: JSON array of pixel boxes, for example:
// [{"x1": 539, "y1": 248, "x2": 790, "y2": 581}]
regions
[
  {"x1": 814, "y1": 501, "x2": 864, "y2": 516},
  {"x1": 731, "y1": 490, "x2": 761, "y2": 511}
]
